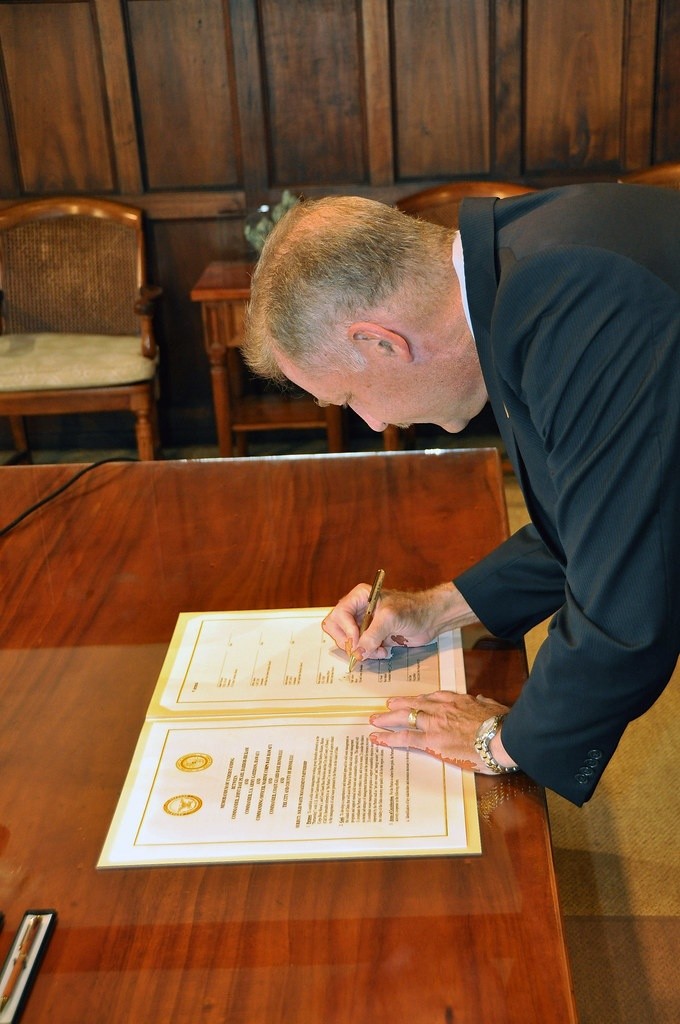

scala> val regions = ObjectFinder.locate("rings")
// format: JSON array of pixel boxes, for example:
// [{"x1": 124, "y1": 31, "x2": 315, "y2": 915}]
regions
[{"x1": 409, "y1": 708, "x2": 423, "y2": 727}]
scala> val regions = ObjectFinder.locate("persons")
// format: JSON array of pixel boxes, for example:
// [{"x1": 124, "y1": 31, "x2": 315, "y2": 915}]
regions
[{"x1": 244, "y1": 182, "x2": 680, "y2": 811}]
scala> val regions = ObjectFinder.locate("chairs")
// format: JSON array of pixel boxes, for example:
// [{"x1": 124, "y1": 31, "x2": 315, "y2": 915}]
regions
[
  {"x1": 382, "y1": 182, "x2": 538, "y2": 453},
  {"x1": 0, "y1": 199, "x2": 164, "y2": 463}
]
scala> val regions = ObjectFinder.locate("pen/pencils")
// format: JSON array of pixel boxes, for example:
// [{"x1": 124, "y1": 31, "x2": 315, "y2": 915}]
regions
[
  {"x1": 0, "y1": 916, "x2": 43, "y2": 1014},
  {"x1": 345, "y1": 568, "x2": 386, "y2": 674}
]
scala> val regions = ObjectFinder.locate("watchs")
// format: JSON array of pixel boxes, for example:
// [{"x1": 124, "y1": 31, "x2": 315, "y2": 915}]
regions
[{"x1": 474, "y1": 712, "x2": 522, "y2": 774}]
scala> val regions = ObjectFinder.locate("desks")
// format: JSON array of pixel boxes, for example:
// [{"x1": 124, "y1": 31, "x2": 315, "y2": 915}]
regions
[
  {"x1": 191, "y1": 261, "x2": 343, "y2": 453},
  {"x1": 0, "y1": 448, "x2": 579, "y2": 1024}
]
